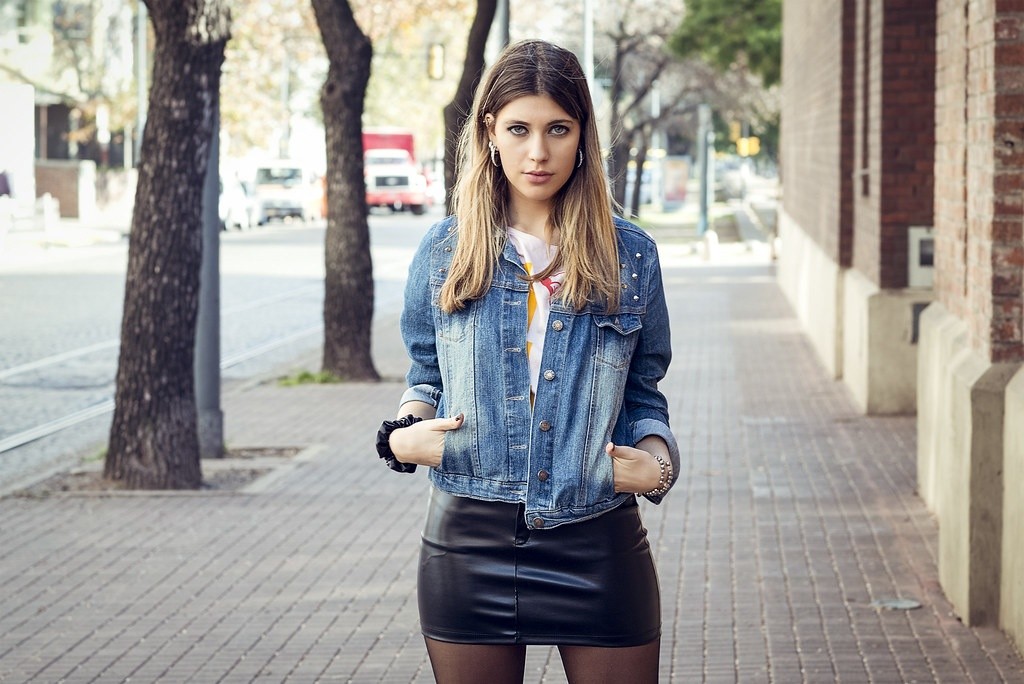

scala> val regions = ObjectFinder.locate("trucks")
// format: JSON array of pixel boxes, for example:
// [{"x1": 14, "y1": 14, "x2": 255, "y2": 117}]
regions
[{"x1": 358, "y1": 127, "x2": 417, "y2": 165}]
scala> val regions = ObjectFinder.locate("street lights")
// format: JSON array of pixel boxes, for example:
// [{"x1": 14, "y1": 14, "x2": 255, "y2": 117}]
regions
[{"x1": 699, "y1": 103, "x2": 711, "y2": 240}]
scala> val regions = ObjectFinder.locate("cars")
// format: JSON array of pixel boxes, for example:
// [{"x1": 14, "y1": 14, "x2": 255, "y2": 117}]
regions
[
  {"x1": 311, "y1": 148, "x2": 431, "y2": 218},
  {"x1": 724, "y1": 157, "x2": 757, "y2": 204},
  {"x1": 600, "y1": 148, "x2": 660, "y2": 208},
  {"x1": 691, "y1": 161, "x2": 730, "y2": 204},
  {"x1": 241, "y1": 160, "x2": 305, "y2": 226}
]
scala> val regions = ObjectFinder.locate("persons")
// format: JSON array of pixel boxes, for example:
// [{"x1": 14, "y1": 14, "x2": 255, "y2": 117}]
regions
[{"x1": 389, "y1": 38, "x2": 680, "y2": 684}]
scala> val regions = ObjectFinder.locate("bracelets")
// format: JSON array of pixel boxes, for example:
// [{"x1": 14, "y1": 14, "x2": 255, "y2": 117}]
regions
[
  {"x1": 645, "y1": 455, "x2": 665, "y2": 495},
  {"x1": 376, "y1": 414, "x2": 423, "y2": 474},
  {"x1": 652, "y1": 460, "x2": 673, "y2": 496}
]
